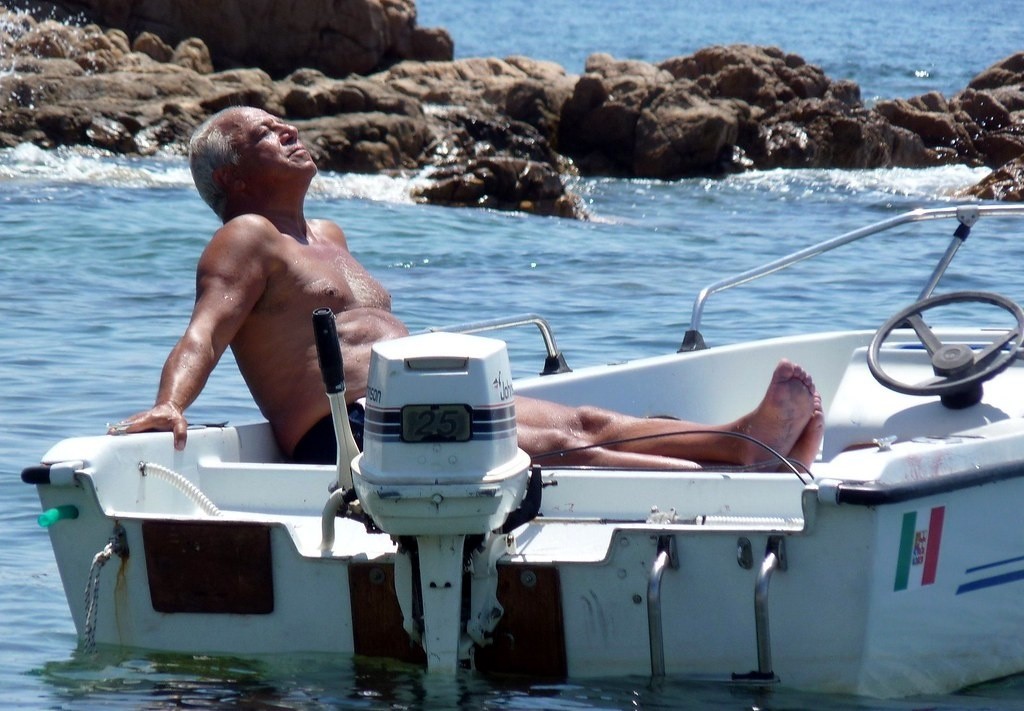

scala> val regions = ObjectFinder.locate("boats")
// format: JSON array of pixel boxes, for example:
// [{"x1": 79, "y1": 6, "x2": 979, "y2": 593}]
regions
[{"x1": 20, "y1": 204, "x2": 1024, "y2": 698}]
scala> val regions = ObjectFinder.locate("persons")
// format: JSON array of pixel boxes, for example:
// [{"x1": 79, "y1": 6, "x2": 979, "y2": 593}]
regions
[{"x1": 107, "y1": 105, "x2": 824, "y2": 475}]
